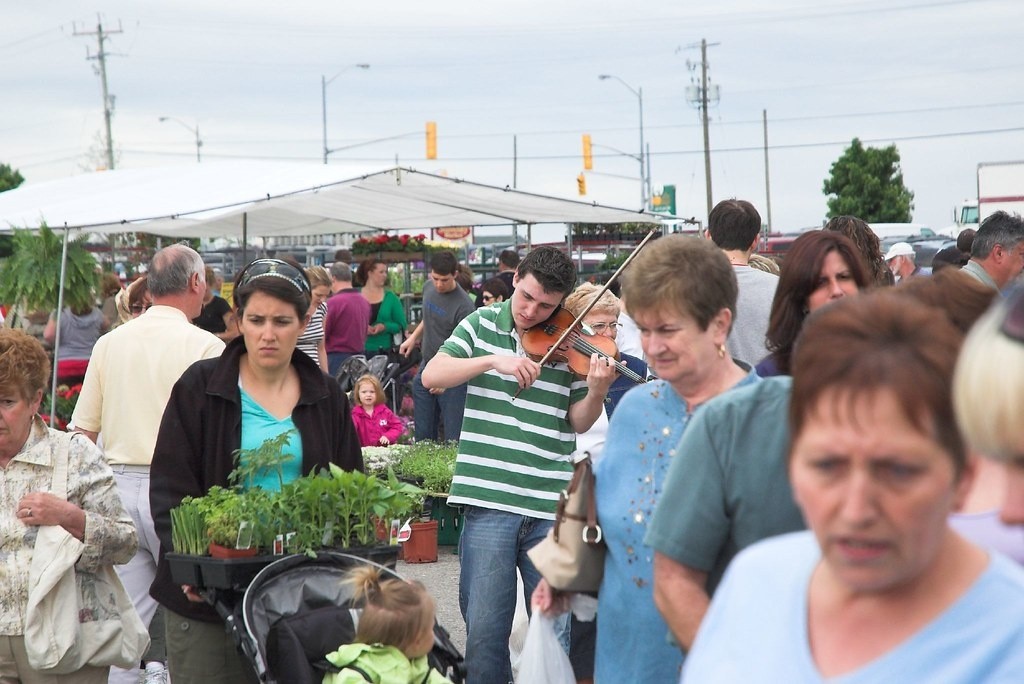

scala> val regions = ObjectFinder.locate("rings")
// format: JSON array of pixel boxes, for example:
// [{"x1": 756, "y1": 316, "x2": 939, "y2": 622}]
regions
[{"x1": 28, "y1": 509, "x2": 32, "y2": 516}]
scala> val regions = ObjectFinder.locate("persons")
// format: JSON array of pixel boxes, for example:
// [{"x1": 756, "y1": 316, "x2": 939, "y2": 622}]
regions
[
  {"x1": 148, "y1": 257, "x2": 364, "y2": 684},
  {"x1": 755, "y1": 212, "x2": 1024, "y2": 375},
  {"x1": 292, "y1": 250, "x2": 409, "y2": 383},
  {"x1": 419, "y1": 246, "x2": 629, "y2": 684},
  {"x1": 352, "y1": 372, "x2": 406, "y2": 451},
  {"x1": 396, "y1": 251, "x2": 524, "y2": 446},
  {"x1": 700, "y1": 193, "x2": 781, "y2": 372},
  {"x1": 42, "y1": 271, "x2": 124, "y2": 398},
  {"x1": 322, "y1": 563, "x2": 457, "y2": 684},
  {"x1": 68, "y1": 241, "x2": 227, "y2": 684},
  {"x1": 187, "y1": 264, "x2": 241, "y2": 343},
  {"x1": 0, "y1": 323, "x2": 138, "y2": 684},
  {"x1": 641, "y1": 287, "x2": 1024, "y2": 684},
  {"x1": 528, "y1": 232, "x2": 763, "y2": 684},
  {"x1": 561, "y1": 280, "x2": 649, "y2": 419}
]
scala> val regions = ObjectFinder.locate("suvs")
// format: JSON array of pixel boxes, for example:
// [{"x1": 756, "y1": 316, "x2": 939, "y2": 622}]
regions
[{"x1": 460, "y1": 242, "x2": 618, "y2": 295}]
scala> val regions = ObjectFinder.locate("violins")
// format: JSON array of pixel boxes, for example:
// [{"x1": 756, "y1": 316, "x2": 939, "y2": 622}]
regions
[{"x1": 521, "y1": 306, "x2": 645, "y2": 383}]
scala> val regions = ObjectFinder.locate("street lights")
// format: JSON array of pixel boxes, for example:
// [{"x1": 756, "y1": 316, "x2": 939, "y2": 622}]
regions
[
  {"x1": 599, "y1": 75, "x2": 645, "y2": 211},
  {"x1": 322, "y1": 63, "x2": 371, "y2": 164},
  {"x1": 159, "y1": 115, "x2": 203, "y2": 164}
]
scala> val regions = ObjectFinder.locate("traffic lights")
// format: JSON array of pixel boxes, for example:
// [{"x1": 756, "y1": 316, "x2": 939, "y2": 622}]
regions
[{"x1": 575, "y1": 174, "x2": 585, "y2": 197}]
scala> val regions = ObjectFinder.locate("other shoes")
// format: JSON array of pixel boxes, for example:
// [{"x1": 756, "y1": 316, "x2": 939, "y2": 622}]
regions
[{"x1": 145, "y1": 668, "x2": 167, "y2": 684}]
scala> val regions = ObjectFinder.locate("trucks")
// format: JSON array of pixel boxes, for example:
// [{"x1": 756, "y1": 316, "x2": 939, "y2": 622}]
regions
[{"x1": 952, "y1": 160, "x2": 1024, "y2": 244}]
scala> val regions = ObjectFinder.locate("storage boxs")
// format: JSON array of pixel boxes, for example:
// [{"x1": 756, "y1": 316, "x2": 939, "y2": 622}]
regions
[{"x1": 431, "y1": 497, "x2": 465, "y2": 545}]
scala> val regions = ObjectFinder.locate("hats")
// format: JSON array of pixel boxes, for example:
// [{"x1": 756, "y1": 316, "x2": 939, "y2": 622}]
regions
[{"x1": 885, "y1": 242, "x2": 915, "y2": 261}]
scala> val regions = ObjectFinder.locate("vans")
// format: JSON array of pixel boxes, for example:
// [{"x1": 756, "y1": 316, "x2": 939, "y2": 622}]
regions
[{"x1": 757, "y1": 224, "x2": 955, "y2": 283}]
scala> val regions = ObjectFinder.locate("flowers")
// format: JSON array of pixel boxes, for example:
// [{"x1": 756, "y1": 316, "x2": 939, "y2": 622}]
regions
[{"x1": 348, "y1": 234, "x2": 428, "y2": 261}]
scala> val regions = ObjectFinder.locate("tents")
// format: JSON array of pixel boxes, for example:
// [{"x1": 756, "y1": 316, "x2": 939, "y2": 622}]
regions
[{"x1": 0, "y1": 155, "x2": 703, "y2": 425}]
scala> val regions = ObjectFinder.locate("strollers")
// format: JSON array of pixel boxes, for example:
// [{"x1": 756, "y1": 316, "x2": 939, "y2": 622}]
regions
[
  {"x1": 200, "y1": 547, "x2": 467, "y2": 684},
  {"x1": 337, "y1": 344, "x2": 420, "y2": 416}
]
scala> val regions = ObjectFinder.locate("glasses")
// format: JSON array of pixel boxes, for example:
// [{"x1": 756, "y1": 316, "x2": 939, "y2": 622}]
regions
[
  {"x1": 239, "y1": 262, "x2": 303, "y2": 287},
  {"x1": 129, "y1": 305, "x2": 152, "y2": 313},
  {"x1": 483, "y1": 296, "x2": 497, "y2": 301}
]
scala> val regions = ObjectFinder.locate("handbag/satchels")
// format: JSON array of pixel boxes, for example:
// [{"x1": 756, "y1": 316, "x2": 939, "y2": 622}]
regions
[
  {"x1": 527, "y1": 458, "x2": 606, "y2": 594},
  {"x1": 24, "y1": 431, "x2": 151, "y2": 673},
  {"x1": 506, "y1": 594, "x2": 600, "y2": 683}
]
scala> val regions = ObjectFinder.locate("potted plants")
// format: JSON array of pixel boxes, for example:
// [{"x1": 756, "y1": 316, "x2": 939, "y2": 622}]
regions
[
  {"x1": 596, "y1": 249, "x2": 631, "y2": 289},
  {"x1": 164, "y1": 428, "x2": 438, "y2": 591},
  {"x1": 565, "y1": 222, "x2": 662, "y2": 244}
]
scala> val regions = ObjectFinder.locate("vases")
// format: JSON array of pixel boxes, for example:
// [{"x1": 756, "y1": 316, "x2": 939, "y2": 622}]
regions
[{"x1": 350, "y1": 251, "x2": 424, "y2": 261}]
